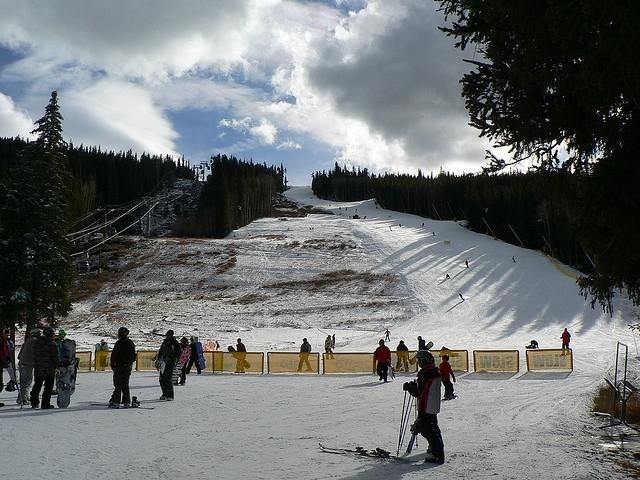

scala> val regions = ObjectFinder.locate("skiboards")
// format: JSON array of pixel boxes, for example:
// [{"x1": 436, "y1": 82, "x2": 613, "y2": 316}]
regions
[
  {"x1": 89, "y1": 398, "x2": 168, "y2": 410},
  {"x1": 318, "y1": 442, "x2": 418, "y2": 463}
]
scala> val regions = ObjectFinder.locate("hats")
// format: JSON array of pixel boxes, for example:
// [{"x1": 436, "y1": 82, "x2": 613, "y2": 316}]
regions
[{"x1": 27, "y1": 326, "x2": 67, "y2": 339}]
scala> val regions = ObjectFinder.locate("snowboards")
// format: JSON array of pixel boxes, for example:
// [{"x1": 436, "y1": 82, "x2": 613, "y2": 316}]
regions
[
  {"x1": 410, "y1": 341, "x2": 433, "y2": 365},
  {"x1": 228, "y1": 346, "x2": 251, "y2": 369}
]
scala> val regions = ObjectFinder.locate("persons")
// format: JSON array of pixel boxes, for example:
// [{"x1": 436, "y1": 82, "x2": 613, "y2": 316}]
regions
[
  {"x1": 233, "y1": 338, "x2": 247, "y2": 373},
  {"x1": 512, "y1": 255, "x2": 516, "y2": 263},
  {"x1": 325, "y1": 335, "x2": 335, "y2": 361},
  {"x1": 394, "y1": 340, "x2": 410, "y2": 373},
  {"x1": 384, "y1": 328, "x2": 391, "y2": 342},
  {"x1": 526, "y1": 339, "x2": 540, "y2": 349},
  {"x1": 560, "y1": 328, "x2": 572, "y2": 357},
  {"x1": 0, "y1": 314, "x2": 219, "y2": 409},
  {"x1": 373, "y1": 339, "x2": 392, "y2": 383},
  {"x1": 439, "y1": 354, "x2": 457, "y2": 400},
  {"x1": 446, "y1": 274, "x2": 451, "y2": 278},
  {"x1": 332, "y1": 334, "x2": 336, "y2": 349},
  {"x1": 402, "y1": 349, "x2": 445, "y2": 465},
  {"x1": 296, "y1": 338, "x2": 314, "y2": 372},
  {"x1": 413, "y1": 336, "x2": 426, "y2": 372},
  {"x1": 466, "y1": 260, "x2": 469, "y2": 268},
  {"x1": 459, "y1": 294, "x2": 465, "y2": 301}
]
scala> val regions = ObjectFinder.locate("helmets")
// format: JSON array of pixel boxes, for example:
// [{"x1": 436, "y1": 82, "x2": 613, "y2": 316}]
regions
[
  {"x1": 442, "y1": 354, "x2": 450, "y2": 361},
  {"x1": 416, "y1": 350, "x2": 435, "y2": 368}
]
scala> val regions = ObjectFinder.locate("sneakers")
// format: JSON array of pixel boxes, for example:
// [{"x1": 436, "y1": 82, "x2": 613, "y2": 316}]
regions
[
  {"x1": 424, "y1": 445, "x2": 444, "y2": 464},
  {"x1": 108, "y1": 395, "x2": 141, "y2": 406},
  {"x1": 171, "y1": 380, "x2": 187, "y2": 386},
  {"x1": 160, "y1": 394, "x2": 174, "y2": 401},
  {"x1": 15, "y1": 396, "x2": 54, "y2": 409}
]
